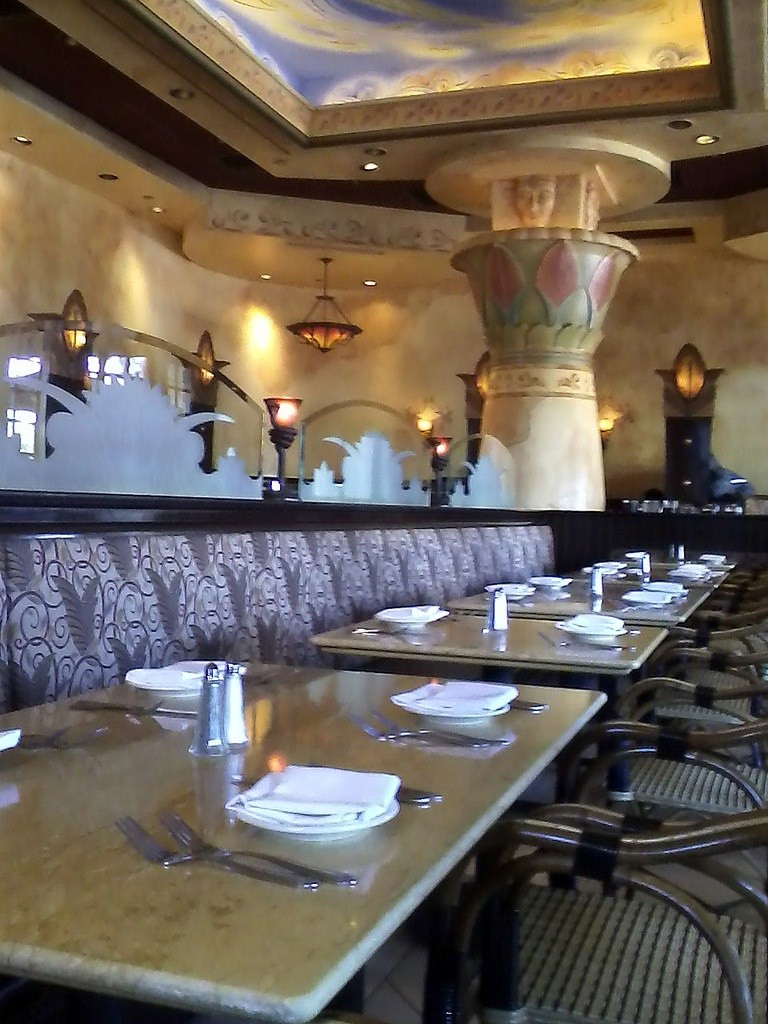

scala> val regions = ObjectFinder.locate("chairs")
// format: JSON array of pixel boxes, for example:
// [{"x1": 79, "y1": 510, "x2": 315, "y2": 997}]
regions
[{"x1": 423, "y1": 568, "x2": 768, "y2": 1024}]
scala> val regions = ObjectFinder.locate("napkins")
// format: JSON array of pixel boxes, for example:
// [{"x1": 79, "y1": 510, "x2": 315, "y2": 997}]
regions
[{"x1": 123, "y1": 552, "x2": 725, "y2": 826}]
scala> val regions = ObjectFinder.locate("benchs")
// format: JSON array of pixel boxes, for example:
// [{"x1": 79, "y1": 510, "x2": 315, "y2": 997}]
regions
[{"x1": 0, "y1": 489, "x2": 555, "y2": 715}]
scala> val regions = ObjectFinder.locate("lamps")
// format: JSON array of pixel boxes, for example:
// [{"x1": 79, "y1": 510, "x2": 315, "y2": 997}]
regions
[
  {"x1": 426, "y1": 437, "x2": 453, "y2": 494},
  {"x1": 415, "y1": 421, "x2": 433, "y2": 439},
  {"x1": 599, "y1": 418, "x2": 614, "y2": 451},
  {"x1": 286, "y1": 257, "x2": 363, "y2": 354},
  {"x1": 28, "y1": 289, "x2": 100, "y2": 380},
  {"x1": 456, "y1": 351, "x2": 491, "y2": 419},
  {"x1": 654, "y1": 343, "x2": 725, "y2": 417},
  {"x1": 264, "y1": 398, "x2": 303, "y2": 485},
  {"x1": 171, "y1": 330, "x2": 231, "y2": 407}
]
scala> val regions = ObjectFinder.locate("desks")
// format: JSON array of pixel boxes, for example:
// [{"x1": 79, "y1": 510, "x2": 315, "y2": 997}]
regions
[
  {"x1": 0, "y1": 660, "x2": 607, "y2": 1024},
  {"x1": 309, "y1": 613, "x2": 670, "y2": 894},
  {"x1": 561, "y1": 566, "x2": 730, "y2": 680},
  {"x1": 446, "y1": 580, "x2": 715, "y2": 792}
]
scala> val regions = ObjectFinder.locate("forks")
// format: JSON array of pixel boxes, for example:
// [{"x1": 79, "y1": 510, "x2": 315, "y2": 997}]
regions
[
  {"x1": 158, "y1": 811, "x2": 359, "y2": 888},
  {"x1": 539, "y1": 633, "x2": 625, "y2": 650},
  {"x1": 369, "y1": 708, "x2": 511, "y2": 747},
  {"x1": 346, "y1": 713, "x2": 491, "y2": 749},
  {"x1": 116, "y1": 815, "x2": 320, "y2": 891},
  {"x1": 69, "y1": 700, "x2": 163, "y2": 715}
]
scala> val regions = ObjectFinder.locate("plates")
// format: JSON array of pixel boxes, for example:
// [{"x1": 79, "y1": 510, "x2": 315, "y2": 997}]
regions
[
  {"x1": 582, "y1": 566, "x2": 618, "y2": 575},
  {"x1": 529, "y1": 576, "x2": 573, "y2": 587},
  {"x1": 128, "y1": 680, "x2": 187, "y2": 690},
  {"x1": 625, "y1": 551, "x2": 646, "y2": 559},
  {"x1": 594, "y1": 561, "x2": 627, "y2": 569},
  {"x1": 622, "y1": 590, "x2": 679, "y2": 604},
  {"x1": 641, "y1": 582, "x2": 683, "y2": 593},
  {"x1": 235, "y1": 796, "x2": 402, "y2": 835},
  {"x1": 374, "y1": 606, "x2": 450, "y2": 623},
  {"x1": 554, "y1": 621, "x2": 628, "y2": 636},
  {"x1": 485, "y1": 583, "x2": 533, "y2": 600},
  {"x1": 401, "y1": 703, "x2": 511, "y2": 718}
]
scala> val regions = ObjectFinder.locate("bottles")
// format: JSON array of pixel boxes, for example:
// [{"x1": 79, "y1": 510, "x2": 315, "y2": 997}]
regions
[
  {"x1": 221, "y1": 661, "x2": 250, "y2": 751},
  {"x1": 642, "y1": 553, "x2": 651, "y2": 573},
  {"x1": 592, "y1": 567, "x2": 603, "y2": 597},
  {"x1": 669, "y1": 543, "x2": 674, "y2": 558},
  {"x1": 678, "y1": 545, "x2": 684, "y2": 560},
  {"x1": 499, "y1": 588, "x2": 509, "y2": 630},
  {"x1": 189, "y1": 662, "x2": 231, "y2": 758},
  {"x1": 493, "y1": 590, "x2": 504, "y2": 630}
]
